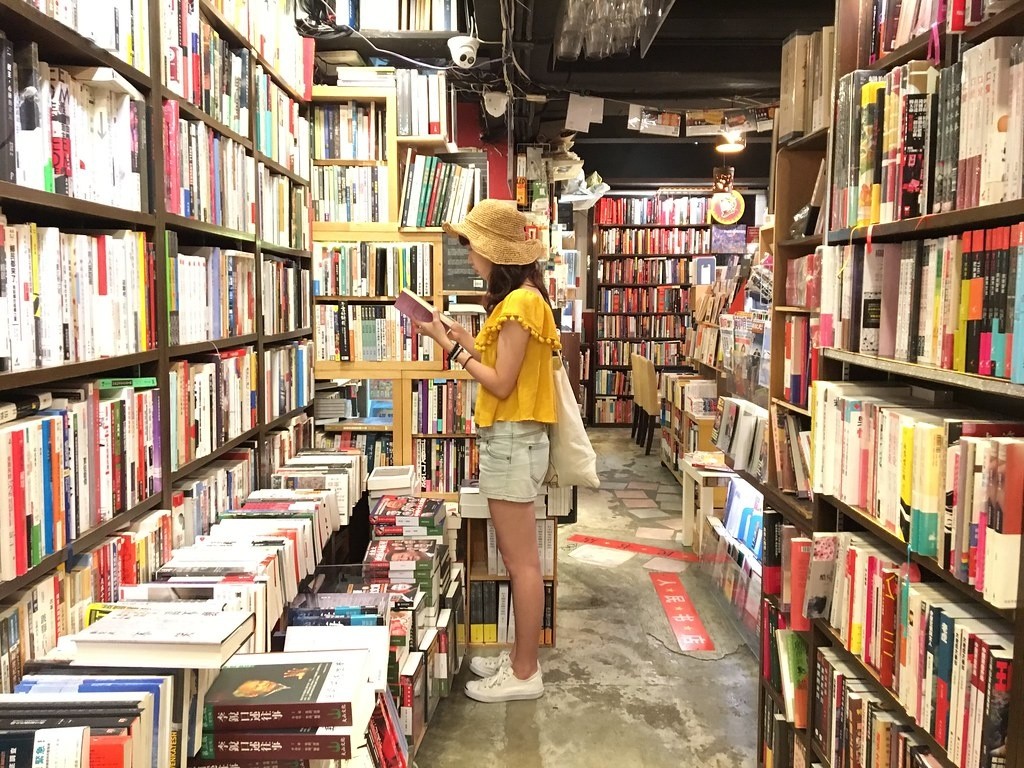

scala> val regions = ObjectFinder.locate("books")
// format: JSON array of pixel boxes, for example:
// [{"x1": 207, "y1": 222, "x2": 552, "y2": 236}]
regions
[
  {"x1": 594, "y1": 1, "x2": 1024, "y2": 768},
  {"x1": 0, "y1": 0, "x2": 591, "y2": 768},
  {"x1": 394, "y1": 286, "x2": 455, "y2": 335}
]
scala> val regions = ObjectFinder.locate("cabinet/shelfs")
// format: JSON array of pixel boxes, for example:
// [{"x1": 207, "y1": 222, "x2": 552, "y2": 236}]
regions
[
  {"x1": 302, "y1": 0, "x2": 559, "y2": 649},
  {"x1": 0, "y1": 0, "x2": 316, "y2": 695},
  {"x1": 586, "y1": 0, "x2": 1024, "y2": 768}
]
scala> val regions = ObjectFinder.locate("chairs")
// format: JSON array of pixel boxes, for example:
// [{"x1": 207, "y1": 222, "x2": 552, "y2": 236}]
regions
[
  {"x1": 630, "y1": 352, "x2": 661, "y2": 444},
  {"x1": 639, "y1": 356, "x2": 662, "y2": 455}
]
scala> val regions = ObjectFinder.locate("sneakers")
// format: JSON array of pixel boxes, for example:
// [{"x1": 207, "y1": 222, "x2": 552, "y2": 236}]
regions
[
  {"x1": 463, "y1": 664, "x2": 544, "y2": 702},
  {"x1": 470, "y1": 650, "x2": 512, "y2": 677}
]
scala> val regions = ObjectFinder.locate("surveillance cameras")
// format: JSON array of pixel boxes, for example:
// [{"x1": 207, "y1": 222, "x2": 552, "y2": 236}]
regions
[
  {"x1": 484, "y1": 91, "x2": 510, "y2": 117},
  {"x1": 448, "y1": 36, "x2": 479, "y2": 69}
]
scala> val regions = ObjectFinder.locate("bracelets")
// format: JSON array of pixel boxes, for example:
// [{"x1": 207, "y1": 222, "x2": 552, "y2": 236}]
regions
[
  {"x1": 462, "y1": 355, "x2": 473, "y2": 369},
  {"x1": 446, "y1": 340, "x2": 463, "y2": 363}
]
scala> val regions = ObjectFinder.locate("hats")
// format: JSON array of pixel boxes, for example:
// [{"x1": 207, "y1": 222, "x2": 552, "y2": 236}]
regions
[{"x1": 440, "y1": 199, "x2": 544, "y2": 265}]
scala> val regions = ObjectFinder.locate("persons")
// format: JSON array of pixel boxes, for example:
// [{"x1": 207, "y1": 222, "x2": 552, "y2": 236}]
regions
[{"x1": 412, "y1": 198, "x2": 561, "y2": 703}]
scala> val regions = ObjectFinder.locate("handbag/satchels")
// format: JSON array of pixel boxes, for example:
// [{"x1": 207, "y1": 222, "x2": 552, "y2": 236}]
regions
[{"x1": 542, "y1": 348, "x2": 601, "y2": 490}]
